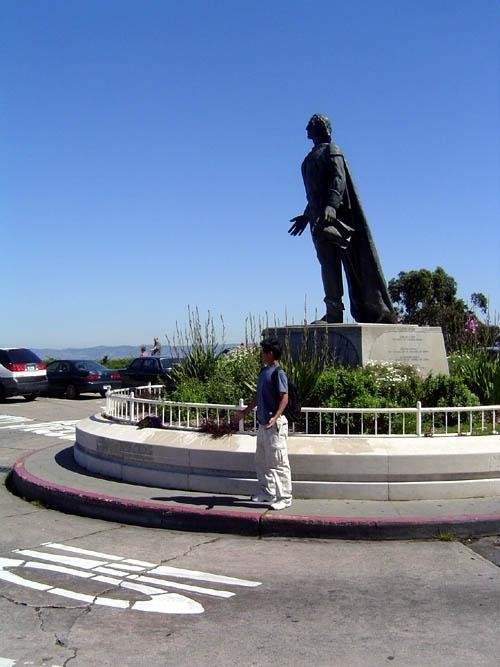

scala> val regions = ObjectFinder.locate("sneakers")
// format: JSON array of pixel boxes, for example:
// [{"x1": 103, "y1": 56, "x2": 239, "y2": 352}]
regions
[
  {"x1": 250, "y1": 493, "x2": 276, "y2": 502},
  {"x1": 270, "y1": 501, "x2": 292, "y2": 510}
]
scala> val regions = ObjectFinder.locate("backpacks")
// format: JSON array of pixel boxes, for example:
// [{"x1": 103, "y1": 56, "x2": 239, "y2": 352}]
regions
[{"x1": 260, "y1": 366, "x2": 300, "y2": 422}]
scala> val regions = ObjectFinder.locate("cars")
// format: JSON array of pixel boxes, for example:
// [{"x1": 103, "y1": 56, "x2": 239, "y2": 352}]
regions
[
  {"x1": 41, "y1": 358, "x2": 122, "y2": 399},
  {"x1": 113, "y1": 355, "x2": 186, "y2": 394},
  {"x1": 474, "y1": 346, "x2": 500, "y2": 362}
]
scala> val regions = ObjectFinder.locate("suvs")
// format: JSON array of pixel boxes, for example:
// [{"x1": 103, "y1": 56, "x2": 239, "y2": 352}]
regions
[{"x1": 0, "y1": 347, "x2": 48, "y2": 404}]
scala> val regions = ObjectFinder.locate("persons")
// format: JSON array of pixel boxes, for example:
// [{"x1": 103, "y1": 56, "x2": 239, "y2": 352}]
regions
[
  {"x1": 150, "y1": 336, "x2": 162, "y2": 358},
  {"x1": 232, "y1": 336, "x2": 293, "y2": 510},
  {"x1": 285, "y1": 112, "x2": 398, "y2": 326},
  {"x1": 139, "y1": 347, "x2": 149, "y2": 357}
]
